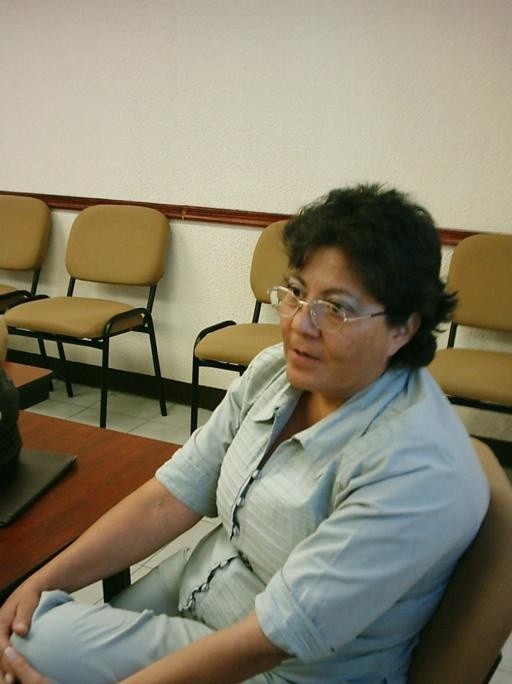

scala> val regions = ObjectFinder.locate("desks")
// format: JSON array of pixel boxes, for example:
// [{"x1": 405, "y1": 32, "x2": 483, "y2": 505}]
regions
[{"x1": 0, "y1": 362, "x2": 190, "y2": 603}]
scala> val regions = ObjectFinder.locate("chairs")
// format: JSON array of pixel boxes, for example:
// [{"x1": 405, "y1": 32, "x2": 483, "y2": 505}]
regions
[
  {"x1": 427, "y1": 230, "x2": 512, "y2": 411},
  {"x1": 409, "y1": 433, "x2": 512, "y2": 682},
  {"x1": 187, "y1": 217, "x2": 292, "y2": 436}
]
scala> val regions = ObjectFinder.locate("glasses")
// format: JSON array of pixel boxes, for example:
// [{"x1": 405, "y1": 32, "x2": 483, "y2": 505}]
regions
[{"x1": 265, "y1": 277, "x2": 392, "y2": 335}]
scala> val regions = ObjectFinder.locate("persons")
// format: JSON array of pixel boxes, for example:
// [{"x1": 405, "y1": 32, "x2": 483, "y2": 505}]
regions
[{"x1": 0, "y1": 182, "x2": 493, "y2": 682}]
